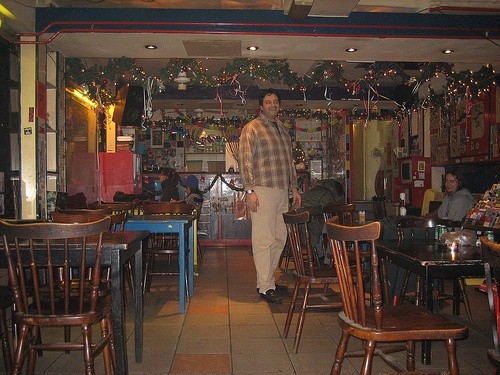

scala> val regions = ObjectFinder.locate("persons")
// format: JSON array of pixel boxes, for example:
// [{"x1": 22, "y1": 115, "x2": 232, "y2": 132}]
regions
[
  {"x1": 238, "y1": 88, "x2": 301, "y2": 303},
  {"x1": 425, "y1": 169, "x2": 473, "y2": 232},
  {"x1": 162, "y1": 174, "x2": 203, "y2": 205},
  {"x1": 301, "y1": 179, "x2": 352, "y2": 245}
]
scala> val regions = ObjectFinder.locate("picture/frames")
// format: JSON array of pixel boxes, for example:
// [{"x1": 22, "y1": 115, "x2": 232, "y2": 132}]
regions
[{"x1": 150, "y1": 128, "x2": 165, "y2": 148}]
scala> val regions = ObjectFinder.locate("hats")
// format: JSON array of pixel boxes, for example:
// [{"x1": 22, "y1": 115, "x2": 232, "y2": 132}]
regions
[{"x1": 182, "y1": 175, "x2": 199, "y2": 188}]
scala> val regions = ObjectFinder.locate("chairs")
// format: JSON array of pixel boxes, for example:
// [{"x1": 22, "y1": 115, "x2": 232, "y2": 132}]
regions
[
  {"x1": 281, "y1": 202, "x2": 472, "y2": 375},
  {"x1": 0, "y1": 200, "x2": 202, "y2": 375}
]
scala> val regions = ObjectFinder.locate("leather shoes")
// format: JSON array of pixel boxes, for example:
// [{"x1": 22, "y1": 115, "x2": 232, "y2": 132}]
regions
[
  {"x1": 259, "y1": 289, "x2": 282, "y2": 304},
  {"x1": 256, "y1": 284, "x2": 288, "y2": 294}
]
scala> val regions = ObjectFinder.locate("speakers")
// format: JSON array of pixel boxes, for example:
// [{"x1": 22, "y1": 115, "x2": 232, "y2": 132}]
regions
[{"x1": 111, "y1": 86, "x2": 147, "y2": 126}]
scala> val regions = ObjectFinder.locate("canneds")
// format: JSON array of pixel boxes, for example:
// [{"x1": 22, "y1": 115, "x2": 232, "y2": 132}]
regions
[
  {"x1": 434, "y1": 224, "x2": 447, "y2": 241},
  {"x1": 484, "y1": 231, "x2": 494, "y2": 242}
]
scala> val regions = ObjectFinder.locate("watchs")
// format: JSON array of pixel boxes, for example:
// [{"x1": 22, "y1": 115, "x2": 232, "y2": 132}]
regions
[{"x1": 246, "y1": 190, "x2": 254, "y2": 193}]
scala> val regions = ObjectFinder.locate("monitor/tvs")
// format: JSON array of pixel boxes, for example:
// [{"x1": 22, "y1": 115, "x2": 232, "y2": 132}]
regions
[{"x1": 401, "y1": 159, "x2": 413, "y2": 184}]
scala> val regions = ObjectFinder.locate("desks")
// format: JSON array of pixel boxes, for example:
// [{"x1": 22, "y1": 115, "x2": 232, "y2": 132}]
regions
[
  {"x1": 112, "y1": 204, "x2": 198, "y2": 266},
  {"x1": 113, "y1": 216, "x2": 197, "y2": 313},
  {"x1": 325, "y1": 210, "x2": 500, "y2": 365},
  {"x1": 0, "y1": 230, "x2": 149, "y2": 375}
]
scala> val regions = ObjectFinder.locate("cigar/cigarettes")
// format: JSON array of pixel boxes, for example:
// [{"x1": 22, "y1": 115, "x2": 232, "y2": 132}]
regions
[{"x1": 300, "y1": 207, "x2": 304, "y2": 208}]
